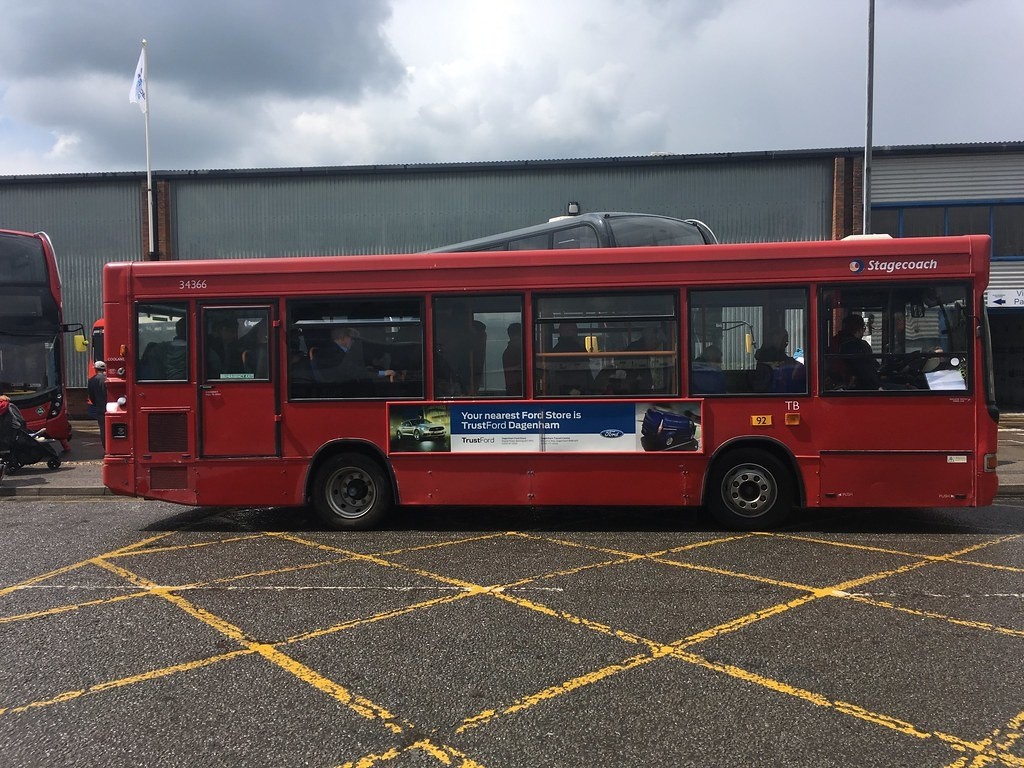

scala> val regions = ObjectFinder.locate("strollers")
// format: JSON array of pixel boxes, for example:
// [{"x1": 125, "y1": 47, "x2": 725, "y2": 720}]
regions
[{"x1": 3, "y1": 402, "x2": 65, "y2": 474}]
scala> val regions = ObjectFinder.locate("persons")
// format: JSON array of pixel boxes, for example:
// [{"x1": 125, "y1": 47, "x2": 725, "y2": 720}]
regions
[
  {"x1": 692, "y1": 345, "x2": 726, "y2": 394},
  {"x1": 210, "y1": 317, "x2": 267, "y2": 378},
  {"x1": 153, "y1": 317, "x2": 222, "y2": 380},
  {"x1": 319, "y1": 326, "x2": 396, "y2": 397},
  {"x1": 548, "y1": 323, "x2": 597, "y2": 396},
  {"x1": 830, "y1": 314, "x2": 887, "y2": 391},
  {"x1": 501, "y1": 322, "x2": 522, "y2": 397},
  {"x1": 89, "y1": 360, "x2": 107, "y2": 448},
  {"x1": 614, "y1": 329, "x2": 664, "y2": 395},
  {"x1": 756, "y1": 327, "x2": 803, "y2": 393}
]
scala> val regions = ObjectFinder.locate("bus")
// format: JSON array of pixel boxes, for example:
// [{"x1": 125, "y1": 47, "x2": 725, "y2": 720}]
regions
[
  {"x1": 402, "y1": 209, "x2": 725, "y2": 250},
  {"x1": 1, "y1": 216, "x2": 92, "y2": 457},
  {"x1": 98, "y1": 222, "x2": 1010, "y2": 532}
]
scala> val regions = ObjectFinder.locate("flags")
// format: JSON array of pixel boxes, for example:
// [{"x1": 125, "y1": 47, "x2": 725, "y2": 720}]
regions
[{"x1": 128, "y1": 47, "x2": 147, "y2": 113}]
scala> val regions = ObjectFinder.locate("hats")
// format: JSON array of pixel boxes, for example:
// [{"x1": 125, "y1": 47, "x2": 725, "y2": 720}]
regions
[{"x1": 92, "y1": 360, "x2": 106, "y2": 370}]
